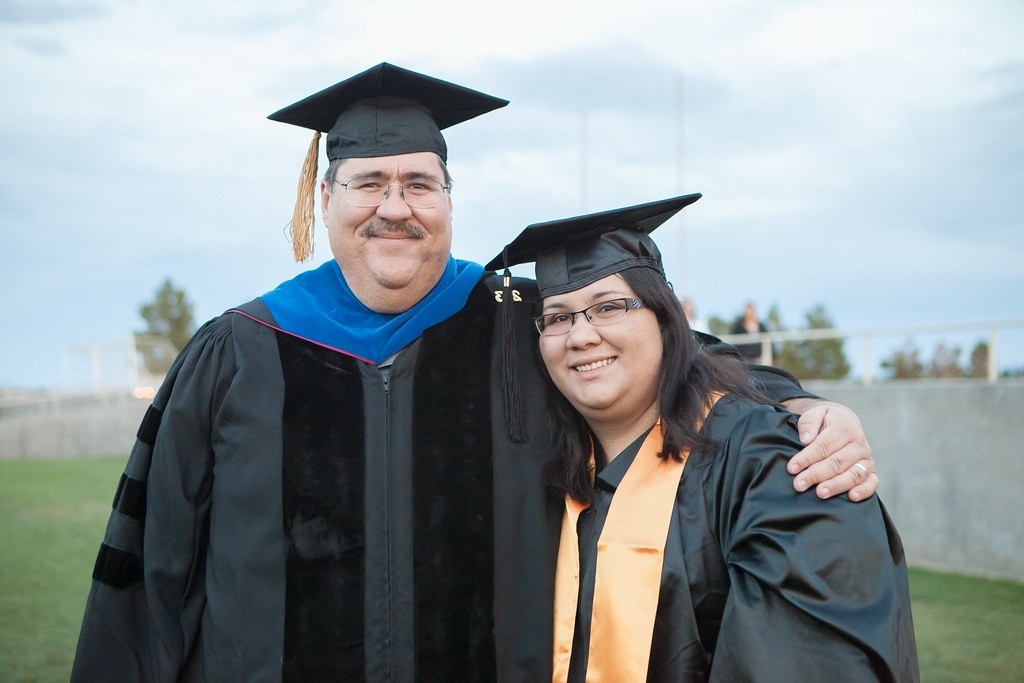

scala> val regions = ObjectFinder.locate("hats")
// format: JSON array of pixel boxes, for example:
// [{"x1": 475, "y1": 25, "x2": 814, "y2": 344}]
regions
[
  {"x1": 482, "y1": 193, "x2": 703, "y2": 444},
  {"x1": 266, "y1": 61, "x2": 512, "y2": 262}
]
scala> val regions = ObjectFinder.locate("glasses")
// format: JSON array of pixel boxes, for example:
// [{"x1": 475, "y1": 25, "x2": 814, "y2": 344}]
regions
[
  {"x1": 529, "y1": 296, "x2": 645, "y2": 338},
  {"x1": 333, "y1": 174, "x2": 451, "y2": 209}
]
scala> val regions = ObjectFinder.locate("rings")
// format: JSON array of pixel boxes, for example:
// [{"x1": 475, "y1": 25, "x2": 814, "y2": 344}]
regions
[{"x1": 855, "y1": 463, "x2": 869, "y2": 478}]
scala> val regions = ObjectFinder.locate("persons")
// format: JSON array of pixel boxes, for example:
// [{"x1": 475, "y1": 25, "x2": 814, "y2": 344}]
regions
[
  {"x1": 70, "y1": 62, "x2": 878, "y2": 683},
  {"x1": 483, "y1": 193, "x2": 920, "y2": 683},
  {"x1": 730, "y1": 305, "x2": 778, "y2": 365},
  {"x1": 680, "y1": 296, "x2": 709, "y2": 333}
]
